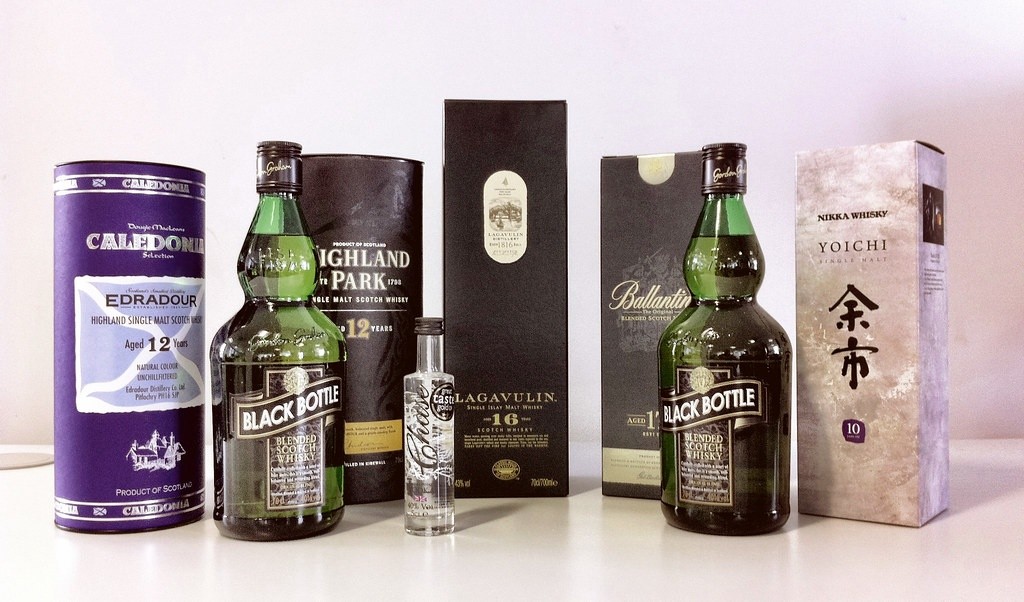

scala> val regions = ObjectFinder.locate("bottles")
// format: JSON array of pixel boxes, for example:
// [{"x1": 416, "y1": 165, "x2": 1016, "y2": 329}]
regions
[
  {"x1": 209, "y1": 141, "x2": 347, "y2": 541},
  {"x1": 403, "y1": 317, "x2": 456, "y2": 537},
  {"x1": 658, "y1": 142, "x2": 793, "y2": 536}
]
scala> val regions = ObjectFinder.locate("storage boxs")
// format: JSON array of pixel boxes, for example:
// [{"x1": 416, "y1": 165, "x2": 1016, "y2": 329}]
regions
[
  {"x1": 442, "y1": 99, "x2": 569, "y2": 497},
  {"x1": 793, "y1": 137, "x2": 951, "y2": 527},
  {"x1": 301, "y1": 153, "x2": 424, "y2": 505},
  {"x1": 600, "y1": 149, "x2": 703, "y2": 499}
]
